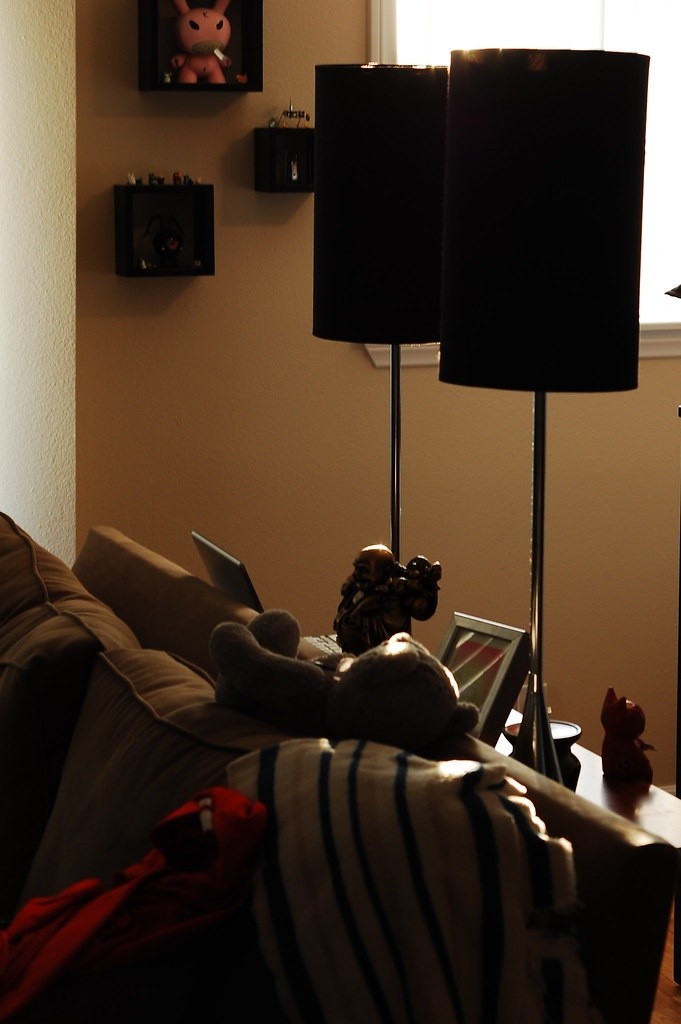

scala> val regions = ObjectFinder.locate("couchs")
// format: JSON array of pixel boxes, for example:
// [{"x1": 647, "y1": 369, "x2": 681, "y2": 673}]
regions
[{"x1": 0, "y1": 509, "x2": 681, "y2": 1024}]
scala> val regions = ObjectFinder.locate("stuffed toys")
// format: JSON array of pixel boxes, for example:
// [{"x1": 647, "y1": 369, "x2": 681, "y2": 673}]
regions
[{"x1": 210, "y1": 610, "x2": 479, "y2": 759}]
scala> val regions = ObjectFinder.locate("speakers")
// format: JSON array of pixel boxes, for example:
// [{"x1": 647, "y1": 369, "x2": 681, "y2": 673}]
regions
[
  {"x1": 314, "y1": 62, "x2": 450, "y2": 345},
  {"x1": 436, "y1": 50, "x2": 652, "y2": 395}
]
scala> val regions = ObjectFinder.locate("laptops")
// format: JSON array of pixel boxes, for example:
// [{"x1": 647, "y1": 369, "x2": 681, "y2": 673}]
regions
[{"x1": 192, "y1": 531, "x2": 344, "y2": 656}]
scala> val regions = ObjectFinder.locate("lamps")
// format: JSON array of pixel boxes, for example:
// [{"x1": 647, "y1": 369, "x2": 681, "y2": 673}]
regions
[
  {"x1": 438, "y1": 48, "x2": 648, "y2": 789},
  {"x1": 313, "y1": 64, "x2": 438, "y2": 562}
]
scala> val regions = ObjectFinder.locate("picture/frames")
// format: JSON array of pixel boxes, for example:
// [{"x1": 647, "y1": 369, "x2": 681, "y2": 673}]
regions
[{"x1": 435, "y1": 615, "x2": 528, "y2": 744}]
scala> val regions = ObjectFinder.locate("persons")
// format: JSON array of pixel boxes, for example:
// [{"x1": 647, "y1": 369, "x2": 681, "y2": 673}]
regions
[{"x1": 332, "y1": 545, "x2": 442, "y2": 656}]
